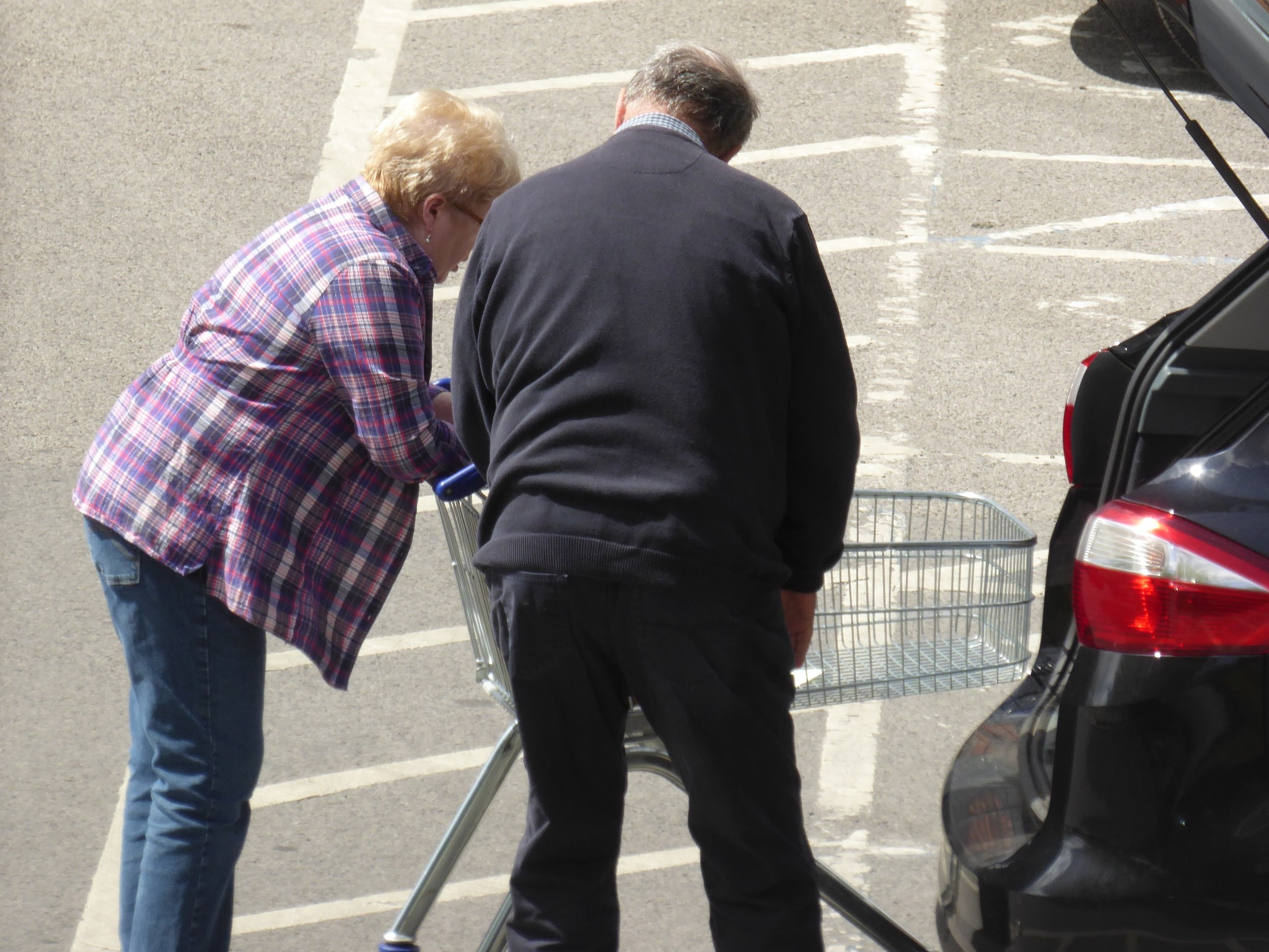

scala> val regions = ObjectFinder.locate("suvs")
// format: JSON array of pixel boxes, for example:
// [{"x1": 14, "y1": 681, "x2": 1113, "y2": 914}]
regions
[{"x1": 932, "y1": 0, "x2": 1269, "y2": 952}]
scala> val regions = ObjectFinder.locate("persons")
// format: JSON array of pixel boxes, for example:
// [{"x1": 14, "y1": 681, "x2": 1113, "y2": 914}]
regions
[
  {"x1": 451, "y1": 42, "x2": 862, "y2": 952},
  {"x1": 72, "y1": 84, "x2": 524, "y2": 952}
]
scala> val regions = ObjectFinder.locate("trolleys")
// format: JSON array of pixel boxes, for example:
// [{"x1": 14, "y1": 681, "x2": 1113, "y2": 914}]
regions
[{"x1": 380, "y1": 376, "x2": 1036, "y2": 952}]
